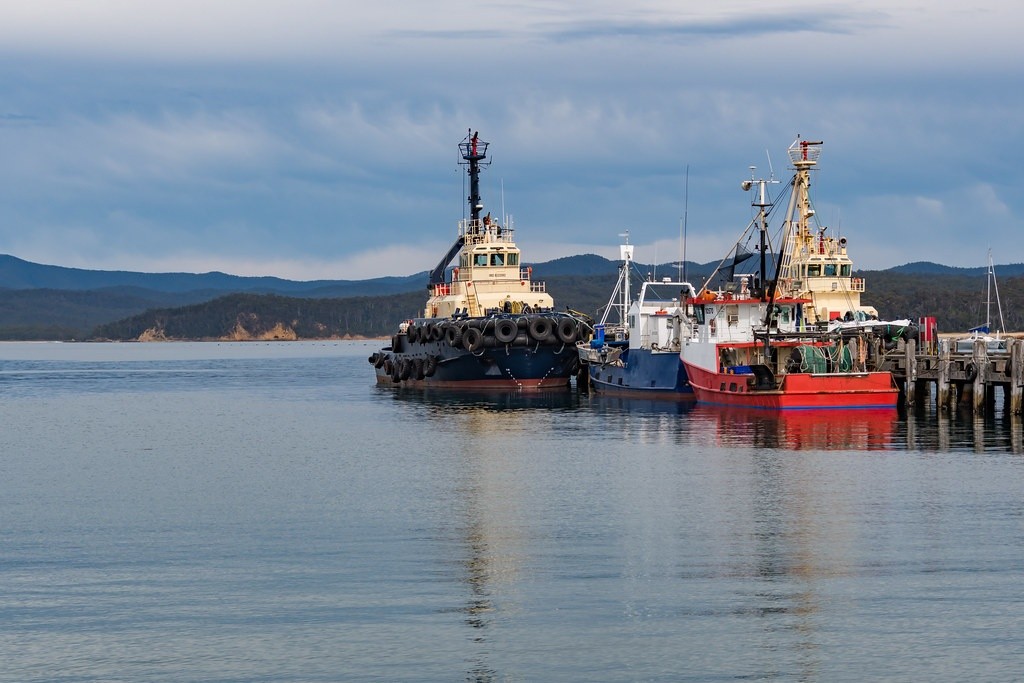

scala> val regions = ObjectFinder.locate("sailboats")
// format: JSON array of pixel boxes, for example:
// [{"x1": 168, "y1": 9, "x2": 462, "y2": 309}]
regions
[{"x1": 953, "y1": 248, "x2": 1014, "y2": 356}]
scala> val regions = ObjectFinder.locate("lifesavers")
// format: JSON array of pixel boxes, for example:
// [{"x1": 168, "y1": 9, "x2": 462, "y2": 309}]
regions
[
  {"x1": 462, "y1": 328, "x2": 483, "y2": 352},
  {"x1": 578, "y1": 322, "x2": 589, "y2": 343},
  {"x1": 494, "y1": 320, "x2": 518, "y2": 343},
  {"x1": 557, "y1": 318, "x2": 577, "y2": 342},
  {"x1": 427, "y1": 322, "x2": 434, "y2": 340},
  {"x1": 371, "y1": 352, "x2": 437, "y2": 383},
  {"x1": 406, "y1": 326, "x2": 416, "y2": 343},
  {"x1": 433, "y1": 326, "x2": 444, "y2": 341},
  {"x1": 416, "y1": 326, "x2": 427, "y2": 344},
  {"x1": 446, "y1": 326, "x2": 463, "y2": 347},
  {"x1": 964, "y1": 363, "x2": 977, "y2": 382},
  {"x1": 879, "y1": 327, "x2": 898, "y2": 349},
  {"x1": 530, "y1": 318, "x2": 552, "y2": 340}
]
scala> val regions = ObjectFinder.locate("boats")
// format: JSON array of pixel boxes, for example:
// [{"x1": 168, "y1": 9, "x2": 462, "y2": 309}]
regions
[
  {"x1": 680, "y1": 133, "x2": 902, "y2": 412},
  {"x1": 368, "y1": 128, "x2": 582, "y2": 402},
  {"x1": 574, "y1": 229, "x2": 694, "y2": 400}
]
